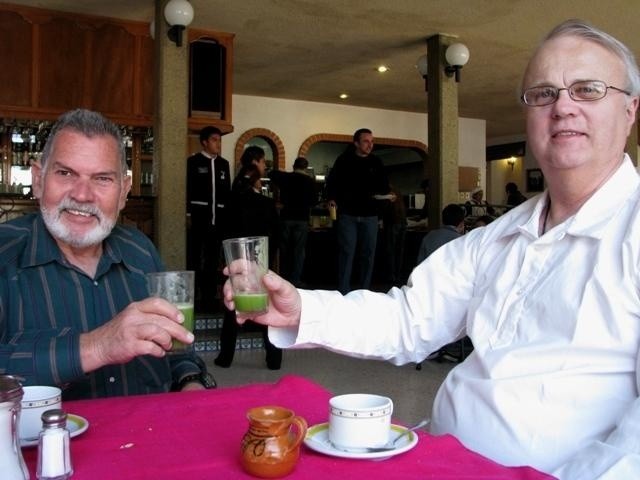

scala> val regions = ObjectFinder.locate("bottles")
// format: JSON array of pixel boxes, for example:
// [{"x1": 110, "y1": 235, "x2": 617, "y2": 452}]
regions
[
  {"x1": 0, "y1": 372, "x2": 33, "y2": 480},
  {"x1": 36, "y1": 408, "x2": 74, "y2": 480},
  {"x1": 13, "y1": 131, "x2": 133, "y2": 164},
  {"x1": 141, "y1": 128, "x2": 154, "y2": 154}
]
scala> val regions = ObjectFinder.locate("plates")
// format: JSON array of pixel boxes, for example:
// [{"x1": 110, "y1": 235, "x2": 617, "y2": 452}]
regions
[
  {"x1": 20, "y1": 411, "x2": 90, "y2": 451},
  {"x1": 305, "y1": 422, "x2": 415, "y2": 461}
]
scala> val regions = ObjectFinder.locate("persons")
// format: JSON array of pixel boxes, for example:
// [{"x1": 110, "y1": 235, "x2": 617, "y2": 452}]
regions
[
  {"x1": 223, "y1": 15, "x2": 640, "y2": 480},
  {"x1": 419, "y1": 199, "x2": 469, "y2": 363},
  {"x1": 268, "y1": 154, "x2": 320, "y2": 291},
  {"x1": 186, "y1": 124, "x2": 233, "y2": 319},
  {"x1": 0, "y1": 107, "x2": 218, "y2": 402},
  {"x1": 329, "y1": 128, "x2": 399, "y2": 298},
  {"x1": 468, "y1": 177, "x2": 530, "y2": 232},
  {"x1": 226, "y1": 143, "x2": 278, "y2": 232}
]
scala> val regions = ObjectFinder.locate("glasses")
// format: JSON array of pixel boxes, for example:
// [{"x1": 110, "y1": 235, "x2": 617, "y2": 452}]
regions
[{"x1": 520, "y1": 81, "x2": 630, "y2": 107}]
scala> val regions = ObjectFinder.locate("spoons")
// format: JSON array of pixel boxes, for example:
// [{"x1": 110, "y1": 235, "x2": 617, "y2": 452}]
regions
[{"x1": 367, "y1": 416, "x2": 432, "y2": 452}]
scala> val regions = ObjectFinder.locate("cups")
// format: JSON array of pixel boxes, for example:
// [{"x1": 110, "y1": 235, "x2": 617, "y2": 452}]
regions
[
  {"x1": 239, "y1": 408, "x2": 307, "y2": 478},
  {"x1": 221, "y1": 233, "x2": 271, "y2": 314},
  {"x1": 327, "y1": 393, "x2": 394, "y2": 453},
  {"x1": 146, "y1": 270, "x2": 198, "y2": 354},
  {"x1": 18, "y1": 385, "x2": 62, "y2": 437}
]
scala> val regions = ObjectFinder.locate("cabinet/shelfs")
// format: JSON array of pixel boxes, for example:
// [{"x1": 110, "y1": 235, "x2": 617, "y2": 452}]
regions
[{"x1": 0, "y1": 1, "x2": 235, "y2": 135}]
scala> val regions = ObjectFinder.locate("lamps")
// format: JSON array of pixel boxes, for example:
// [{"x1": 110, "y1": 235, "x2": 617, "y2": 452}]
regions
[
  {"x1": 164, "y1": 0, "x2": 194, "y2": 47},
  {"x1": 445, "y1": 43, "x2": 470, "y2": 82},
  {"x1": 418, "y1": 55, "x2": 428, "y2": 91}
]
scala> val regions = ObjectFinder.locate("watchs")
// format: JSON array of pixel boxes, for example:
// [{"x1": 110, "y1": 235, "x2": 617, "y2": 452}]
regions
[{"x1": 176, "y1": 370, "x2": 219, "y2": 390}]
